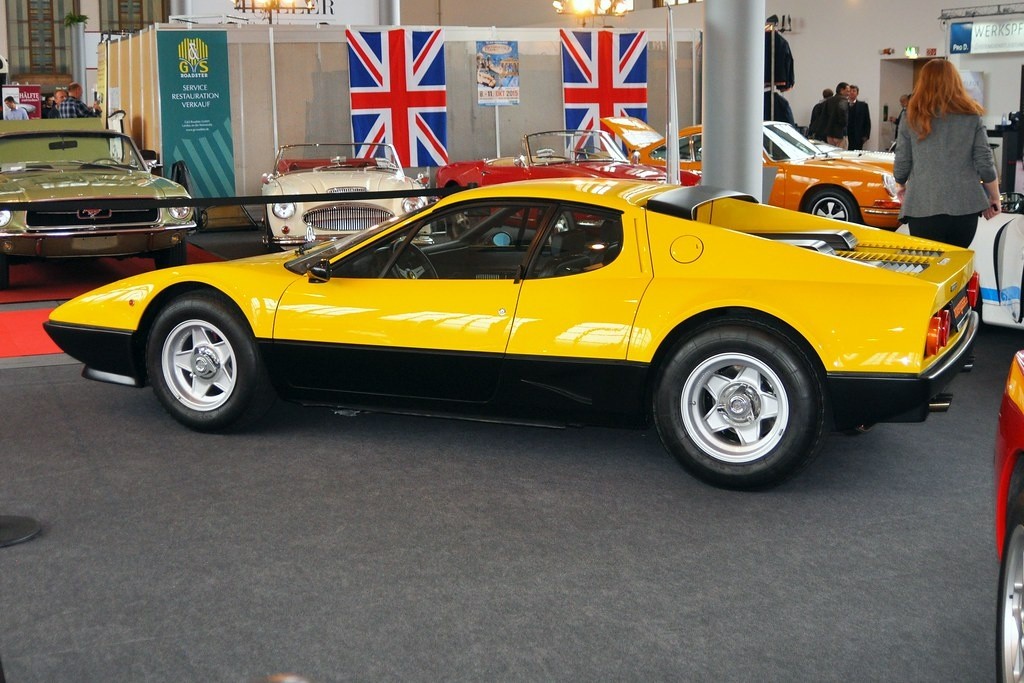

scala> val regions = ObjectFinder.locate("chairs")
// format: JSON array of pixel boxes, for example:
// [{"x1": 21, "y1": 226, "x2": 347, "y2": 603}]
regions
[
  {"x1": 536, "y1": 229, "x2": 590, "y2": 279},
  {"x1": 590, "y1": 220, "x2": 622, "y2": 268}
]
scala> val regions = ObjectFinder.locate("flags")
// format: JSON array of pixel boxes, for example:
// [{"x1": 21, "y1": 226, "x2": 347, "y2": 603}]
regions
[
  {"x1": 560, "y1": 28, "x2": 649, "y2": 158},
  {"x1": 345, "y1": 28, "x2": 448, "y2": 166}
]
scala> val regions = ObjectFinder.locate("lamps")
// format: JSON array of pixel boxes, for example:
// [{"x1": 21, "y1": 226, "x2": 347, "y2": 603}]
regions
[
  {"x1": 551, "y1": 0, "x2": 630, "y2": 18},
  {"x1": 232, "y1": 0, "x2": 315, "y2": 14}
]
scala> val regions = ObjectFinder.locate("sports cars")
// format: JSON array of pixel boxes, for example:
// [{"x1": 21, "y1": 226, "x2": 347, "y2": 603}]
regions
[{"x1": 42, "y1": 179, "x2": 984, "y2": 493}]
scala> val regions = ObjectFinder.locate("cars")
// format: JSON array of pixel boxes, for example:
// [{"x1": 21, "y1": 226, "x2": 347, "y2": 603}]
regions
[
  {"x1": 0, "y1": 117, "x2": 197, "y2": 291},
  {"x1": 436, "y1": 130, "x2": 701, "y2": 233},
  {"x1": 994, "y1": 351, "x2": 1024, "y2": 681},
  {"x1": 262, "y1": 142, "x2": 434, "y2": 251},
  {"x1": 589, "y1": 117, "x2": 905, "y2": 231}
]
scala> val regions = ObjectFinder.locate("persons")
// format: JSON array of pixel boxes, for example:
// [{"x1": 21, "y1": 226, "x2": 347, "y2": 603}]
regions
[
  {"x1": 805, "y1": 83, "x2": 871, "y2": 151},
  {"x1": 894, "y1": 58, "x2": 1002, "y2": 370},
  {"x1": 3, "y1": 95, "x2": 28, "y2": 120},
  {"x1": 890, "y1": 94, "x2": 907, "y2": 139},
  {"x1": 40, "y1": 83, "x2": 102, "y2": 119}
]
